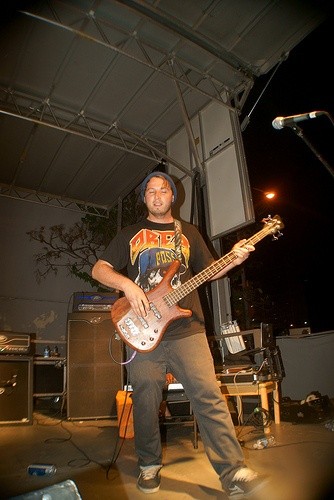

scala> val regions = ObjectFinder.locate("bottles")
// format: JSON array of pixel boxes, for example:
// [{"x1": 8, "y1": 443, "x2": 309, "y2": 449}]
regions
[
  {"x1": 44, "y1": 344, "x2": 49, "y2": 357},
  {"x1": 53, "y1": 346, "x2": 56, "y2": 353}
]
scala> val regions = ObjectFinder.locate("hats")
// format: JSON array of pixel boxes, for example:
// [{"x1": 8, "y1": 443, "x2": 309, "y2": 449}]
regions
[{"x1": 141, "y1": 172, "x2": 177, "y2": 203}]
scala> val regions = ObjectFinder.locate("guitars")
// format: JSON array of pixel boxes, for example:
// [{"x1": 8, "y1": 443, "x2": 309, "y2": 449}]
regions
[{"x1": 110, "y1": 214, "x2": 285, "y2": 353}]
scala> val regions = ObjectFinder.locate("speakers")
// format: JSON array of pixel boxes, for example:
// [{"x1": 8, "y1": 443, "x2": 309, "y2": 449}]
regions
[
  {"x1": 66, "y1": 311, "x2": 124, "y2": 422},
  {"x1": 0, "y1": 354, "x2": 33, "y2": 426}
]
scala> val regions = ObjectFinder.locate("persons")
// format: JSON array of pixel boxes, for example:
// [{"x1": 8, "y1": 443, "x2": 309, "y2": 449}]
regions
[{"x1": 91, "y1": 172, "x2": 268, "y2": 500}]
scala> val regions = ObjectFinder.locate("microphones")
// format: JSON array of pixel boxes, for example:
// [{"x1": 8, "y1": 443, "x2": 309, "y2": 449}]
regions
[{"x1": 272, "y1": 110, "x2": 324, "y2": 130}]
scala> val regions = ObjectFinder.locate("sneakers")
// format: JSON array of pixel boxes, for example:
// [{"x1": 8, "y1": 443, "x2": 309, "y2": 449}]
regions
[
  {"x1": 227, "y1": 468, "x2": 272, "y2": 500},
  {"x1": 136, "y1": 464, "x2": 163, "y2": 493}
]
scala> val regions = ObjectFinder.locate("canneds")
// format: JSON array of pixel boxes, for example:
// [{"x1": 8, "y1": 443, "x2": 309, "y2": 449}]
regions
[{"x1": 27, "y1": 463, "x2": 57, "y2": 476}]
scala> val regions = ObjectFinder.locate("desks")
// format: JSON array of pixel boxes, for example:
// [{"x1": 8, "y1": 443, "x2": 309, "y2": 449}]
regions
[{"x1": 219, "y1": 379, "x2": 280, "y2": 435}]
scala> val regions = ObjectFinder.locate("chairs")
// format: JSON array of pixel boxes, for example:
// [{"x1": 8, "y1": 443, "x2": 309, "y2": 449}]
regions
[{"x1": 159, "y1": 389, "x2": 199, "y2": 450}]
